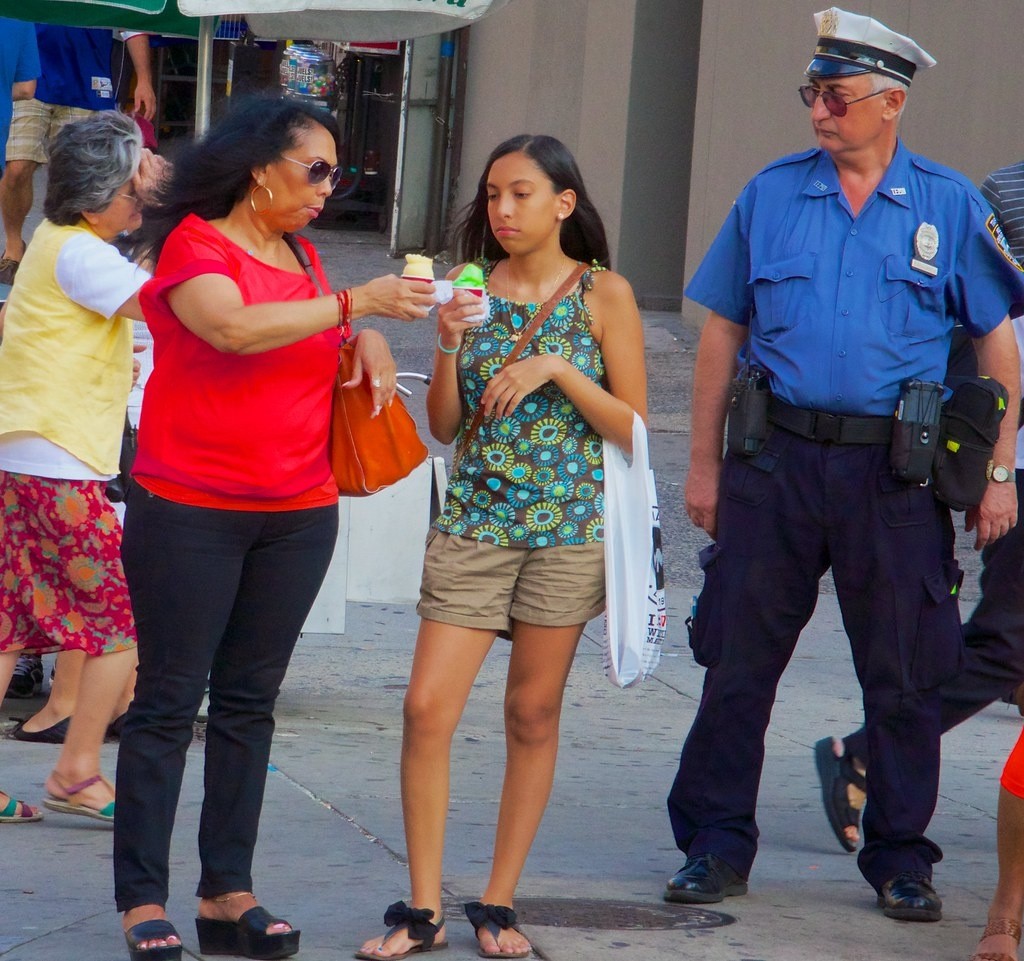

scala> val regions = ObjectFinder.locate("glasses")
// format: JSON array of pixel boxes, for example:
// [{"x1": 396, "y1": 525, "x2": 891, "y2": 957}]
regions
[
  {"x1": 797, "y1": 84, "x2": 897, "y2": 118},
  {"x1": 278, "y1": 153, "x2": 343, "y2": 191},
  {"x1": 113, "y1": 191, "x2": 145, "y2": 207}
]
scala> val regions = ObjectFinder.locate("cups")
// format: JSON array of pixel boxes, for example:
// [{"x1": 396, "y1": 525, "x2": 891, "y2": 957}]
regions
[
  {"x1": 453, "y1": 288, "x2": 482, "y2": 297},
  {"x1": 402, "y1": 277, "x2": 432, "y2": 283}
]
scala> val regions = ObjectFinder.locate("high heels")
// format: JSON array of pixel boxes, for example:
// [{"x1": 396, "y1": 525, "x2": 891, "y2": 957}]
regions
[
  {"x1": 123, "y1": 919, "x2": 184, "y2": 961},
  {"x1": 812, "y1": 733, "x2": 869, "y2": 853},
  {"x1": 194, "y1": 905, "x2": 302, "y2": 960}
]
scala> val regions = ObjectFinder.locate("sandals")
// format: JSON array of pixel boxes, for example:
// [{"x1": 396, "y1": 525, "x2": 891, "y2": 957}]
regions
[
  {"x1": 0, "y1": 789, "x2": 44, "y2": 823},
  {"x1": 41, "y1": 766, "x2": 117, "y2": 822},
  {"x1": 970, "y1": 916, "x2": 1021, "y2": 961},
  {"x1": 353, "y1": 901, "x2": 453, "y2": 961},
  {"x1": 463, "y1": 900, "x2": 533, "y2": 958}
]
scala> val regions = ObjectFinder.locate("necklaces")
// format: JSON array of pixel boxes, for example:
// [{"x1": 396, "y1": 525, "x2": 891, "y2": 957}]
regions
[{"x1": 505, "y1": 255, "x2": 566, "y2": 342}]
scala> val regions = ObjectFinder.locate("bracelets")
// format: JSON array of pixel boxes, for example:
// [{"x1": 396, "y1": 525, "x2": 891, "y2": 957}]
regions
[
  {"x1": 438, "y1": 333, "x2": 461, "y2": 354},
  {"x1": 333, "y1": 286, "x2": 353, "y2": 328}
]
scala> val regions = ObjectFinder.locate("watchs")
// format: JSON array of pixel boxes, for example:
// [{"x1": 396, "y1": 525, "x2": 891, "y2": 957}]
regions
[{"x1": 990, "y1": 464, "x2": 1017, "y2": 483}]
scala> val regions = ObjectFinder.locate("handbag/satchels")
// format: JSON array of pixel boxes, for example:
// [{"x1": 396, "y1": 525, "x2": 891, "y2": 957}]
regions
[
  {"x1": 297, "y1": 453, "x2": 450, "y2": 634},
  {"x1": 931, "y1": 320, "x2": 1010, "y2": 516},
  {"x1": 282, "y1": 231, "x2": 429, "y2": 498},
  {"x1": 599, "y1": 410, "x2": 668, "y2": 691}
]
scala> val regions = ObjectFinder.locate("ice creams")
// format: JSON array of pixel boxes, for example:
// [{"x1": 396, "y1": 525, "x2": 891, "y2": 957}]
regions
[
  {"x1": 453, "y1": 264, "x2": 485, "y2": 289},
  {"x1": 401, "y1": 254, "x2": 433, "y2": 278}
]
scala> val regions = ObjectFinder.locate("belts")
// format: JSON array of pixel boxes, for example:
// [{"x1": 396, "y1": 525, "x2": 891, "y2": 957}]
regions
[{"x1": 761, "y1": 397, "x2": 897, "y2": 447}]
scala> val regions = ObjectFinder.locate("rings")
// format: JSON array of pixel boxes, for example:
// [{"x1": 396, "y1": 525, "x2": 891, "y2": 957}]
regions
[{"x1": 372, "y1": 378, "x2": 381, "y2": 388}]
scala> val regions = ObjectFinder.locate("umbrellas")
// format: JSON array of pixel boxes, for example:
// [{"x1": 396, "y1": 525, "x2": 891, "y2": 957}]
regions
[{"x1": 0, "y1": 0, "x2": 222, "y2": 148}]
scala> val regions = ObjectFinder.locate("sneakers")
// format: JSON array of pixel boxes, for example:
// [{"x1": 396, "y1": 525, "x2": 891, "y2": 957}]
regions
[
  {"x1": 5, "y1": 651, "x2": 45, "y2": 699},
  {"x1": 0, "y1": 238, "x2": 27, "y2": 310}
]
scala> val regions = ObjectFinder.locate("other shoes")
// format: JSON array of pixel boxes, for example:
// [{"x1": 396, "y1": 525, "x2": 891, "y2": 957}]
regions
[
  {"x1": 102, "y1": 710, "x2": 129, "y2": 743},
  {"x1": 3, "y1": 706, "x2": 73, "y2": 743}
]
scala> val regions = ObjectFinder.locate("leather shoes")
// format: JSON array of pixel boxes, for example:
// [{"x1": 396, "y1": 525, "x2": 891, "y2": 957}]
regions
[
  {"x1": 875, "y1": 871, "x2": 944, "y2": 922},
  {"x1": 662, "y1": 851, "x2": 750, "y2": 904}
]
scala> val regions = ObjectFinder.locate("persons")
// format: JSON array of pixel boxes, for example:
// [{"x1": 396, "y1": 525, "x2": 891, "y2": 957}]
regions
[
  {"x1": 811, "y1": 159, "x2": 1024, "y2": 852},
  {"x1": 353, "y1": 131, "x2": 650, "y2": 961},
  {"x1": 665, "y1": 7, "x2": 1024, "y2": 923},
  {"x1": 113, "y1": 91, "x2": 441, "y2": 961},
  {"x1": 1, "y1": 18, "x2": 188, "y2": 826},
  {"x1": 968, "y1": 727, "x2": 1024, "y2": 961}
]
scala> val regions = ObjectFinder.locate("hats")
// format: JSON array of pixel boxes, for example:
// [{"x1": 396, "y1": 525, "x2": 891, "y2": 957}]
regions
[
  {"x1": 124, "y1": 111, "x2": 160, "y2": 149},
  {"x1": 802, "y1": 6, "x2": 938, "y2": 91}
]
